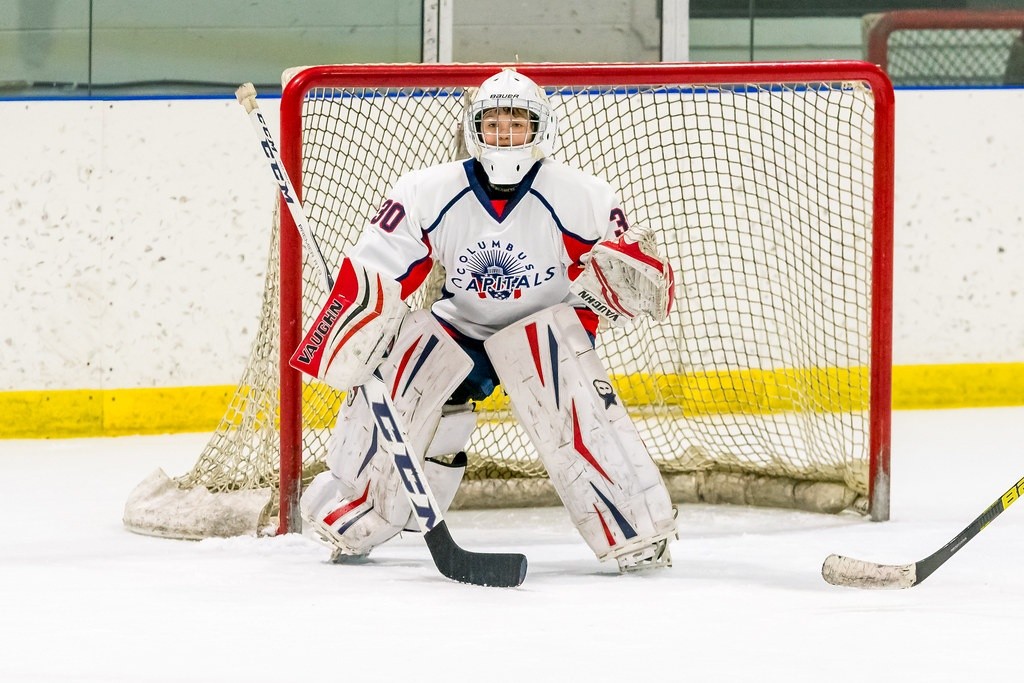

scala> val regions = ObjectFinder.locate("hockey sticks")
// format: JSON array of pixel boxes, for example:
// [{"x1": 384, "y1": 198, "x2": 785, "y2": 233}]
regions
[
  {"x1": 823, "y1": 478, "x2": 1024, "y2": 587},
  {"x1": 232, "y1": 82, "x2": 529, "y2": 588}
]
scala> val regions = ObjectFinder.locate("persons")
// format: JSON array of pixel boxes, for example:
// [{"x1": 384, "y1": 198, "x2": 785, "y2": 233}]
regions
[{"x1": 288, "y1": 70, "x2": 681, "y2": 572}]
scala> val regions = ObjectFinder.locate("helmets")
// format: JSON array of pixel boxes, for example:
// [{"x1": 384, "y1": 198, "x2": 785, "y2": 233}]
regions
[{"x1": 463, "y1": 68, "x2": 557, "y2": 184}]
